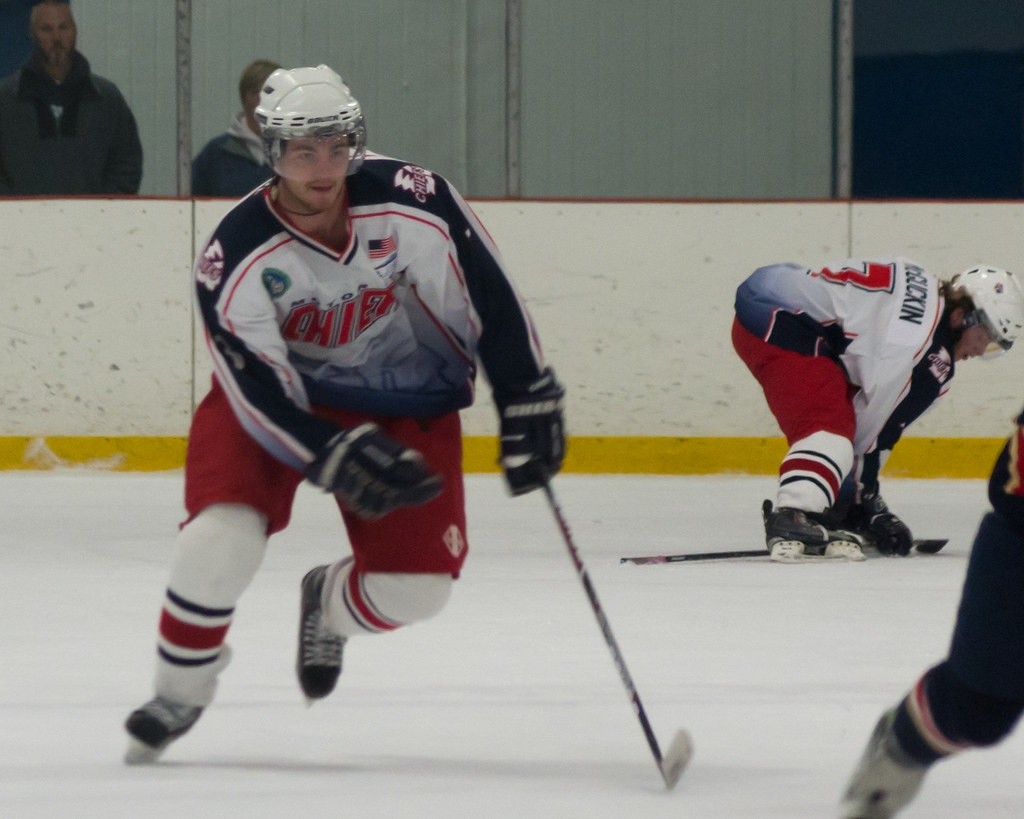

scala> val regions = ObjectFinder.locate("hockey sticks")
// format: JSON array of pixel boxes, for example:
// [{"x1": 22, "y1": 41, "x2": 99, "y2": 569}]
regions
[
  {"x1": 618, "y1": 538, "x2": 950, "y2": 566},
  {"x1": 533, "y1": 433, "x2": 695, "y2": 792}
]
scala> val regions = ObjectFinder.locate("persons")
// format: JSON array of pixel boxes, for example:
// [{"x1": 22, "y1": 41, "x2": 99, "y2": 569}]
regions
[
  {"x1": 2, "y1": 2, "x2": 146, "y2": 194},
  {"x1": 186, "y1": 61, "x2": 290, "y2": 198},
  {"x1": 119, "y1": 61, "x2": 571, "y2": 747},
  {"x1": 730, "y1": 261, "x2": 1021, "y2": 565},
  {"x1": 842, "y1": 401, "x2": 1023, "y2": 819}
]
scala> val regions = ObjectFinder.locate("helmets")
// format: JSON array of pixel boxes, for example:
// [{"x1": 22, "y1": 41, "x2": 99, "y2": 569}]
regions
[
  {"x1": 255, "y1": 62, "x2": 366, "y2": 175},
  {"x1": 948, "y1": 266, "x2": 1023, "y2": 361}
]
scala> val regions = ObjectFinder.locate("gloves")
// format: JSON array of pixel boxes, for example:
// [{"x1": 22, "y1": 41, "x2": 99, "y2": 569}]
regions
[
  {"x1": 499, "y1": 404, "x2": 568, "y2": 496},
  {"x1": 309, "y1": 421, "x2": 444, "y2": 520}
]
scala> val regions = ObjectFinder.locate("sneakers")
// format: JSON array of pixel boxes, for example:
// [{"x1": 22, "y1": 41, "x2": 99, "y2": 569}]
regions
[
  {"x1": 841, "y1": 704, "x2": 932, "y2": 819},
  {"x1": 124, "y1": 694, "x2": 203, "y2": 764},
  {"x1": 297, "y1": 567, "x2": 350, "y2": 708},
  {"x1": 760, "y1": 500, "x2": 864, "y2": 562}
]
